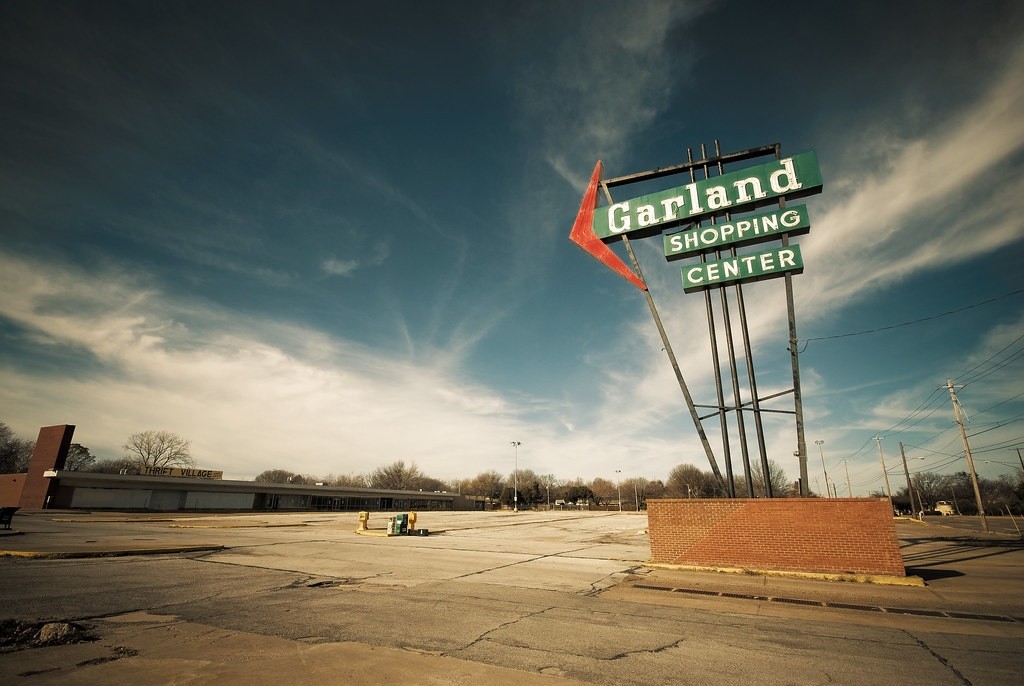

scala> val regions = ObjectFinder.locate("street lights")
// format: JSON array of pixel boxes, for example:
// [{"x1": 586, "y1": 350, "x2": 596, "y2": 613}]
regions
[
  {"x1": 904, "y1": 456, "x2": 925, "y2": 519},
  {"x1": 508, "y1": 441, "x2": 521, "y2": 513},
  {"x1": 815, "y1": 439, "x2": 831, "y2": 498},
  {"x1": 615, "y1": 469, "x2": 622, "y2": 512}
]
